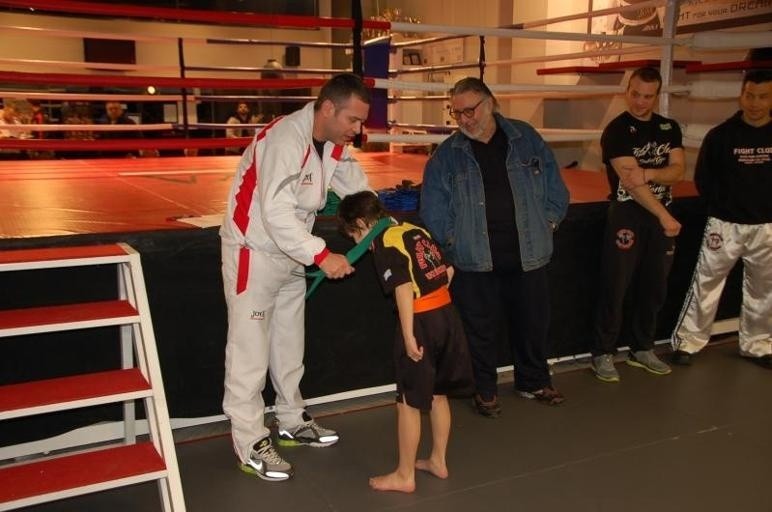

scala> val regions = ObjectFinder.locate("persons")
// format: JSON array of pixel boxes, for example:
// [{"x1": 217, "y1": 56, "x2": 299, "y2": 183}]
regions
[
  {"x1": 419, "y1": 77, "x2": 571, "y2": 419},
  {"x1": 138, "y1": 147, "x2": 160, "y2": 156},
  {"x1": 411, "y1": 54, "x2": 419, "y2": 64},
  {"x1": 224, "y1": 102, "x2": 264, "y2": 155},
  {"x1": 667, "y1": 66, "x2": 772, "y2": 372},
  {"x1": 337, "y1": 190, "x2": 478, "y2": 493},
  {"x1": 404, "y1": 55, "x2": 411, "y2": 64},
  {"x1": 217, "y1": 72, "x2": 374, "y2": 483},
  {"x1": 588, "y1": 66, "x2": 687, "y2": 384},
  {"x1": 92, "y1": 101, "x2": 144, "y2": 156},
  {"x1": 184, "y1": 146, "x2": 198, "y2": 156},
  {"x1": 0, "y1": 96, "x2": 34, "y2": 160}
]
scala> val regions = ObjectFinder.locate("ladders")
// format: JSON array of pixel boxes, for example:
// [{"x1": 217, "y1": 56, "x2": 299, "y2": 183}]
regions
[{"x1": 0, "y1": 241, "x2": 186, "y2": 512}]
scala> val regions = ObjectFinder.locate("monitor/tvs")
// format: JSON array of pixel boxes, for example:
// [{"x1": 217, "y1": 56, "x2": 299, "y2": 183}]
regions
[{"x1": 83, "y1": 37, "x2": 135, "y2": 71}]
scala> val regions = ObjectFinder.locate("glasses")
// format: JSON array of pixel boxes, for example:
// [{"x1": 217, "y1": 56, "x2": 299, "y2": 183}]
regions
[{"x1": 448, "y1": 100, "x2": 483, "y2": 121}]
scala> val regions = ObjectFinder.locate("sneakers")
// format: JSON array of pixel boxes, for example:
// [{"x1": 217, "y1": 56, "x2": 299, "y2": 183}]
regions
[
  {"x1": 276, "y1": 420, "x2": 340, "y2": 447},
  {"x1": 674, "y1": 350, "x2": 691, "y2": 365},
  {"x1": 238, "y1": 438, "x2": 294, "y2": 481},
  {"x1": 514, "y1": 385, "x2": 567, "y2": 406},
  {"x1": 589, "y1": 354, "x2": 620, "y2": 382},
  {"x1": 748, "y1": 354, "x2": 771, "y2": 369},
  {"x1": 471, "y1": 389, "x2": 501, "y2": 417},
  {"x1": 626, "y1": 350, "x2": 671, "y2": 375}
]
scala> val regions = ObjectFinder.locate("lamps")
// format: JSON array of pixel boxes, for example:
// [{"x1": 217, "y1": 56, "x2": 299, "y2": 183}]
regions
[{"x1": 261, "y1": 27, "x2": 284, "y2": 79}]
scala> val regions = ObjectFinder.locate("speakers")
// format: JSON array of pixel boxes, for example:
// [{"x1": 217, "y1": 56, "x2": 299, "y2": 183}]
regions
[{"x1": 286, "y1": 46, "x2": 300, "y2": 66}]
